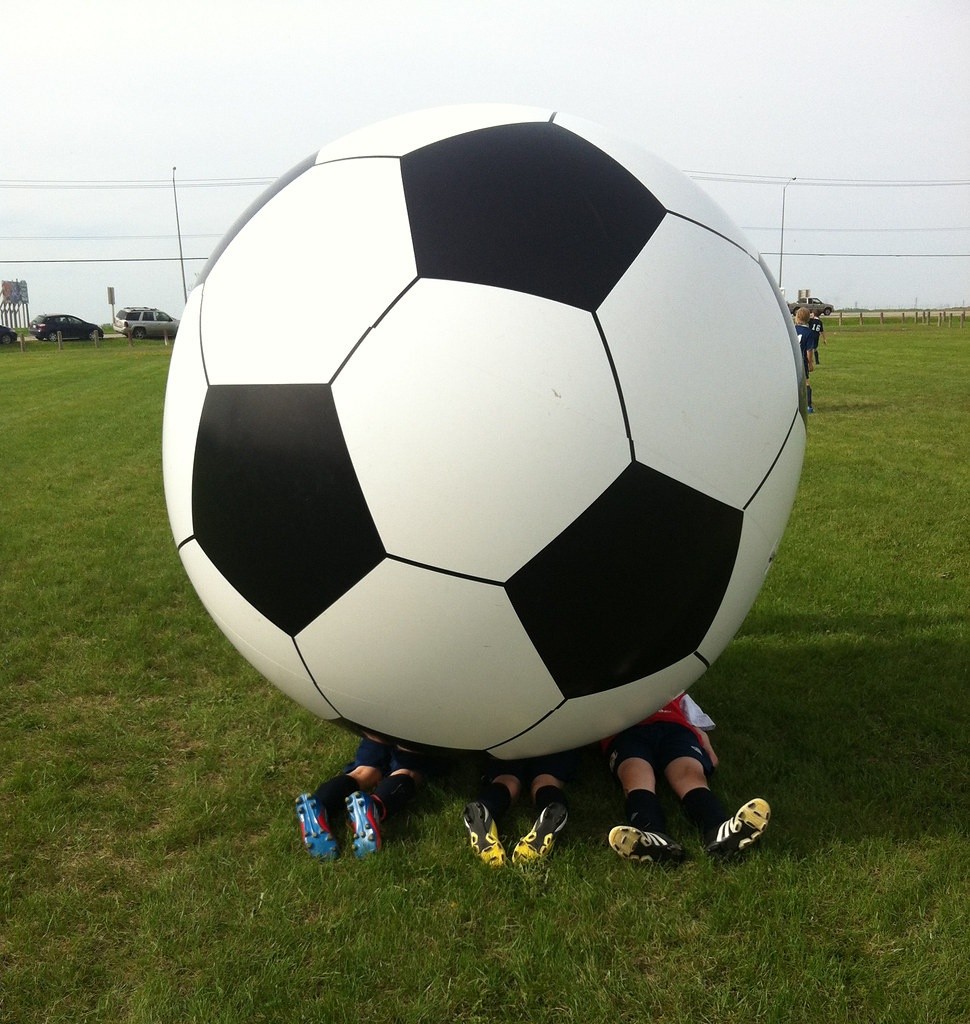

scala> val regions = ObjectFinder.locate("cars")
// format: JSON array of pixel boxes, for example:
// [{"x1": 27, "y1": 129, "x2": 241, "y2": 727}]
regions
[
  {"x1": 0, "y1": 325, "x2": 18, "y2": 345},
  {"x1": 27, "y1": 313, "x2": 104, "y2": 343}
]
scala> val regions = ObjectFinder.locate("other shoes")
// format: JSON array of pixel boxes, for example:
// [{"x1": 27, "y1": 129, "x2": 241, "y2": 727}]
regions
[{"x1": 808, "y1": 406, "x2": 814, "y2": 413}]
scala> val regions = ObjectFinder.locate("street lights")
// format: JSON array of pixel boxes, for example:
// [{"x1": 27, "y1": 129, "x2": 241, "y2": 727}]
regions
[
  {"x1": 170, "y1": 165, "x2": 187, "y2": 304},
  {"x1": 778, "y1": 175, "x2": 798, "y2": 288}
]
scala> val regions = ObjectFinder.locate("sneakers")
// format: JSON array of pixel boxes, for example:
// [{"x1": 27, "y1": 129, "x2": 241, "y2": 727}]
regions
[
  {"x1": 608, "y1": 826, "x2": 687, "y2": 871},
  {"x1": 345, "y1": 791, "x2": 382, "y2": 859},
  {"x1": 463, "y1": 802, "x2": 508, "y2": 870},
  {"x1": 705, "y1": 797, "x2": 771, "y2": 867},
  {"x1": 512, "y1": 802, "x2": 568, "y2": 868},
  {"x1": 295, "y1": 793, "x2": 340, "y2": 862}
]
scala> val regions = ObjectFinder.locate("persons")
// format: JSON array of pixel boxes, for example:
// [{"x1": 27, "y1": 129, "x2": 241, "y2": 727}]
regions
[
  {"x1": 461, "y1": 755, "x2": 568, "y2": 869},
  {"x1": 807, "y1": 310, "x2": 827, "y2": 366},
  {"x1": 602, "y1": 691, "x2": 772, "y2": 870},
  {"x1": 795, "y1": 306, "x2": 815, "y2": 414},
  {"x1": 294, "y1": 740, "x2": 429, "y2": 862}
]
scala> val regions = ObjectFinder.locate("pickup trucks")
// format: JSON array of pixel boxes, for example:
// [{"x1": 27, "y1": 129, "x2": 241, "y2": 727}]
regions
[{"x1": 787, "y1": 296, "x2": 834, "y2": 317}]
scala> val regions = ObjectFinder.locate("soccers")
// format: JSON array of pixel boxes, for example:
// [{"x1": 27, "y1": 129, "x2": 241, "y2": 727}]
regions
[{"x1": 155, "y1": 96, "x2": 818, "y2": 766}]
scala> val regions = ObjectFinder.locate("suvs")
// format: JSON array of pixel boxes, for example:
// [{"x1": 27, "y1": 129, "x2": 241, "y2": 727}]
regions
[{"x1": 113, "y1": 305, "x2": 181, "y2": 340}]
